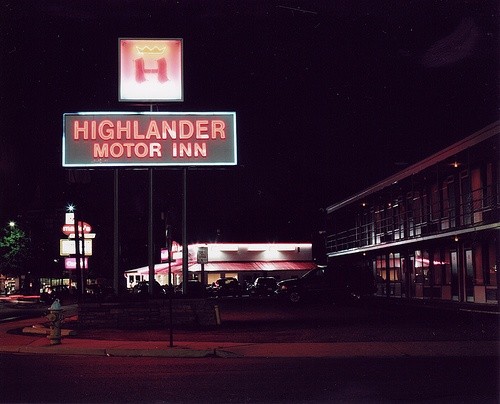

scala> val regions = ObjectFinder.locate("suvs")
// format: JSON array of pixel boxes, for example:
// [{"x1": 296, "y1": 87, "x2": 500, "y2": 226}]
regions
[{"x1": 274, "y1": 266, "x2": 378, "y2": 308}]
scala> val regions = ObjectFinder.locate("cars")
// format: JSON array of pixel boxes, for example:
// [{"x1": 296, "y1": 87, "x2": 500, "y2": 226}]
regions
[
  {"x1": 138, "y1": 281, "x2": 161, "y2": 296},
  {"x1": 253, "y1": 277, "x2": 278, "y2": 296},
  {"x1": 212, "y1": 277, "x2": 239, "y2": 297},
  {"x1": 41, "y1": 285, "x2": 76, "y2": 303}
]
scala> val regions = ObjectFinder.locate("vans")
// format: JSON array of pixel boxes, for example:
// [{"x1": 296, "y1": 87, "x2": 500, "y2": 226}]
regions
[{"x1": 175, "y1": 279, "x2": 199, "y2": 297}]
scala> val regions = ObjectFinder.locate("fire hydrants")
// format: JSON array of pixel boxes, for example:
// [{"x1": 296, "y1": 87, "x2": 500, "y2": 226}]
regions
[{"x1": 46, "y1": 299, "x2": 65, "y2": 345}]
